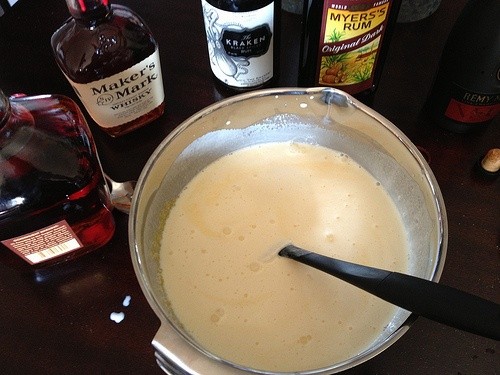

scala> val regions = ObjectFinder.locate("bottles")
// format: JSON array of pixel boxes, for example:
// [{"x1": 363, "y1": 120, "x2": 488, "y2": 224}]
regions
[
  {"x1": 298, "y1": 0, "x2": 402, "y2": 107},
  {"x1": 422, "y1": 1, "x2": 500, "y2": 135},
  {"x1": 50, "y1": 0, "x2": 165, "y2": 138},
  {"x1": 0, "y1": 89, "x2": 115, "y2": 271},
  {"x1": 201, "y1": 0, "x2": 276, "y2": 98}
]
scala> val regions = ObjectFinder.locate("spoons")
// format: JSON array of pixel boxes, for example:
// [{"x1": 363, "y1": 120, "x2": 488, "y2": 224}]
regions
[{"x1": 103, "y1": 171, "x2": 137, "y2": 215}]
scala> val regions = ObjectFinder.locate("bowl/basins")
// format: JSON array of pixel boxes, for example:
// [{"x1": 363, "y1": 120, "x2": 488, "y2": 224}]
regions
[{"x1": 128, "y1": 87, "x2": 449, "y2": 374}]
